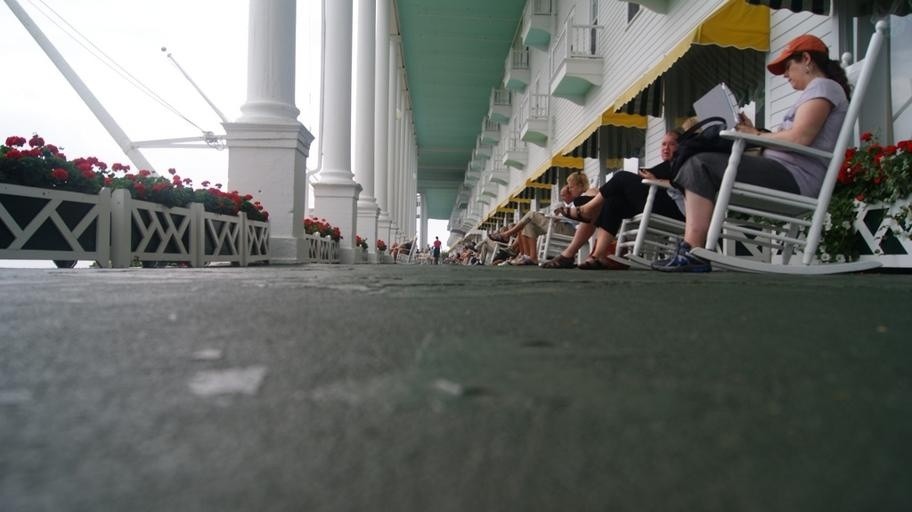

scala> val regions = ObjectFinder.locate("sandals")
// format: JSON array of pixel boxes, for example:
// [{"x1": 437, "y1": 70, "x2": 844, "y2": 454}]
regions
[
  {"x1": 456, "y1": 233, "x2": 537, "y2": 266},
  {"x1": 559, "y1": 207, "x2": 591, "y2": 224},
  {"x1": 542, "y1": 254, "x2": 608, "y2": 270}
]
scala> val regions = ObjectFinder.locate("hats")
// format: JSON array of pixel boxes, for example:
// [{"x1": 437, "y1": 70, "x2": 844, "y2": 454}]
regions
[{"x1": 767, "y1": 34, "x2": 828, "y2": 75}]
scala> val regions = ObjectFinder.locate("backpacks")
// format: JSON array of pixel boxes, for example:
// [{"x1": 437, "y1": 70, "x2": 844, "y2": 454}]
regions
[{"x1": 669, "y1": 117, "x2": 727, "y2": 189}]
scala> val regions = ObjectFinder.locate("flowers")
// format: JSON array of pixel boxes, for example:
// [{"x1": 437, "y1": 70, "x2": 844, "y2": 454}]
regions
[
  {"x1": 1, "y1": 132, "x2": 271, "y2": 221},
  {"x1": 836, "y1": 130, "x2": 910, "y2": 198},
  {"x1": 353, "y1": 234, "x2": 388, "y2": 251},
  {"x1": 304, "y1": 215, "x2": 344, "y2": 244}
]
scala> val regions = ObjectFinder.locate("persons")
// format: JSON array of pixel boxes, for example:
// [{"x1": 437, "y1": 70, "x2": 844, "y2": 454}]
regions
[{"x1": 432, "y1": 236, "x2": 441, "y2": 264}]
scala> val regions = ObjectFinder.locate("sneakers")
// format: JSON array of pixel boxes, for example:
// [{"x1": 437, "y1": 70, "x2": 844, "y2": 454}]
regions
[{"x1": 651, "y1": 238, "x2": 712, "y2": 272}]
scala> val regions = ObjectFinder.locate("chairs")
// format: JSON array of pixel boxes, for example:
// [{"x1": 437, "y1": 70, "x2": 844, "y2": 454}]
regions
[
  {"x1": 686, "y1": 21, "x2": 885, "y2": 271},
  {"x1": 526, "y1": 178, "x2": 596, "y2": 266},
  {"x1": 608, "y1": 179, "x2": 723, "y2": 269}
]
